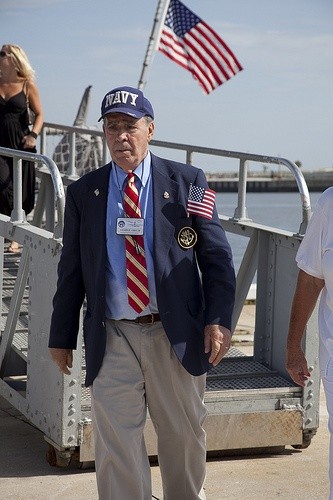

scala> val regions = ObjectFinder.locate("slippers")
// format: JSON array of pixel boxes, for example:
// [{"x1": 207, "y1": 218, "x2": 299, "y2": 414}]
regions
[{"x1": 7, "y1": 243, "x2": 19, "y2": 253}]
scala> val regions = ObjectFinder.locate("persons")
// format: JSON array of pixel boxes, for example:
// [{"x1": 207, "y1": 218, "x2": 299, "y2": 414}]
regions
[
  {"x1": 286, "y1": 186, "x2": 333, "y2": 500},
  {"x1": 48, "y1": 87, "x2": 237, "y2": 500},
  {"x1": 0, "y1": 45, "x2": 43, "y2": 254}
]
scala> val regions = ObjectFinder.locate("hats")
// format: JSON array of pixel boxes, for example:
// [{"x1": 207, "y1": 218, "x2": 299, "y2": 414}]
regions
[{"x1": 97, "y1": 86, "x2": 156, "y2": 121}]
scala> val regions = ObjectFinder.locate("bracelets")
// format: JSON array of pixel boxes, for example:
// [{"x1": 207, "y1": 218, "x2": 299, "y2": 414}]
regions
[{"x1": 29, "y1": 131, "x2": 38, "y2": 139}]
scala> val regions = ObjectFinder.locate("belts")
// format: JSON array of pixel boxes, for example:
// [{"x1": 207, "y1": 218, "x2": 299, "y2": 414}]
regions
[{"x1": 120, "y1": 313, "x2": 160, "y2": 325}]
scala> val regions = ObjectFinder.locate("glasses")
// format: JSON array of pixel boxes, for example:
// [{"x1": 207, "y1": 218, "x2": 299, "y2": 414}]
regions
[{"x1": 0, "y1": 51, "x2": 6, "y2": 57}]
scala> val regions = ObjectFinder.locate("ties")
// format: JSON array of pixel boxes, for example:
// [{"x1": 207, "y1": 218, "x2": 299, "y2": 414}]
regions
[{"x1": 122, "y1": 171, "x2": 149, "y2": 315}]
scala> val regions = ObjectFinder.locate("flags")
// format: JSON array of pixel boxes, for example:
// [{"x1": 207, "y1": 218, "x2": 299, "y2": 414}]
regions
[{"x1": 156, "y1": 0, "x2": 243, "y2": 95}]
